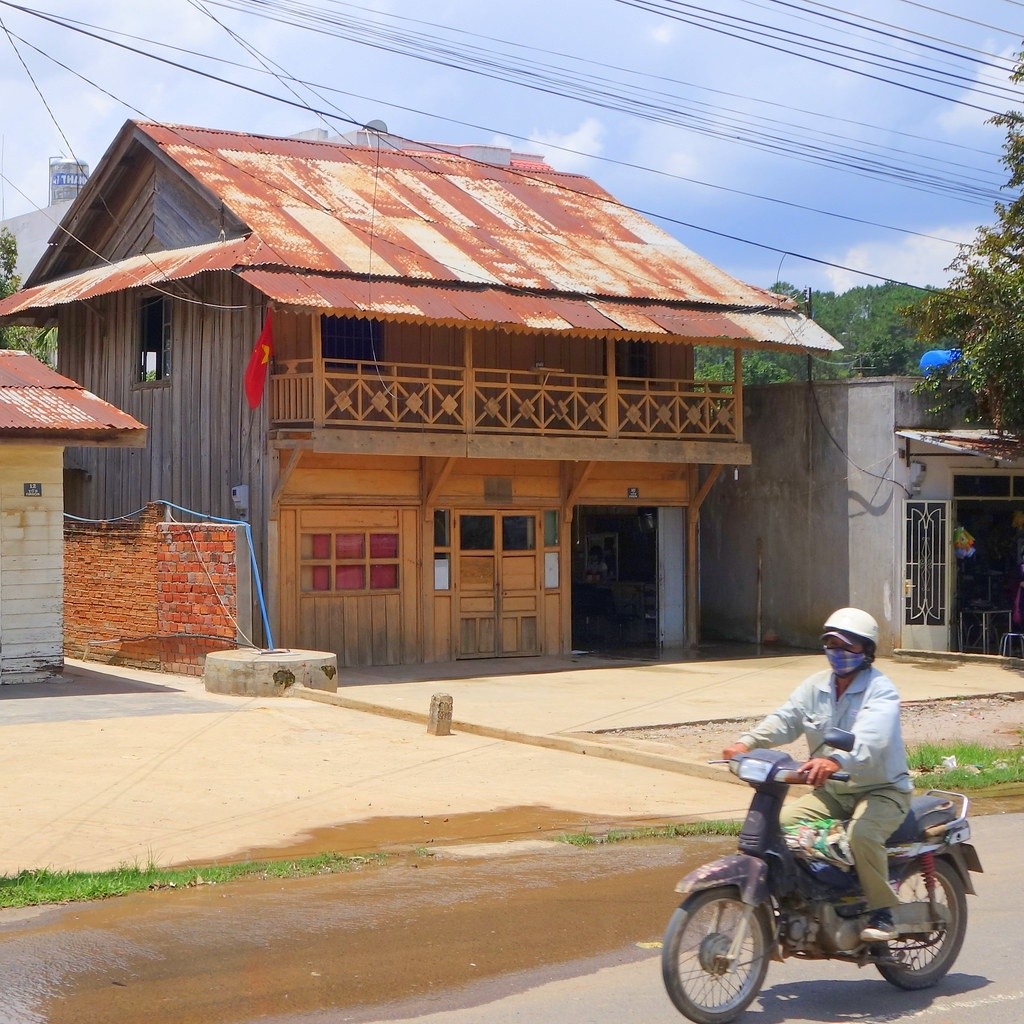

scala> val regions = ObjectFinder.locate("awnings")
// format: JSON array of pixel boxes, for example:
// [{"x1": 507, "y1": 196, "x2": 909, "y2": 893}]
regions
[
  {"x1": 0, "y1": 231, "x2": 844, "y2": 356},
  {"x1": 894, "y1": 424, "x2": 1024, "y2": 462},
  {"x1": 0, "y1": 348, "x2": 149, "y2": 449}
]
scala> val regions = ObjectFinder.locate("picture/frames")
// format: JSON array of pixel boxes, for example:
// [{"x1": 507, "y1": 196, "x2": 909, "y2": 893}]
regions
[{"x1": 584, "y1": 532, "x2": 619, "y2": 582}]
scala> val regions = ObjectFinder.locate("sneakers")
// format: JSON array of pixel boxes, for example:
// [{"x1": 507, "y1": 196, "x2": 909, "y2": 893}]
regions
[{"x1": 860, "y1": 912, "x2": 899, "y2": 941}]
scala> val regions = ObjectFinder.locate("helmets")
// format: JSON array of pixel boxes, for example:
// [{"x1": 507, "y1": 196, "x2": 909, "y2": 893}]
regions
[{"x1": 822, "y1": 608, "x2": 878, "y2": 648}]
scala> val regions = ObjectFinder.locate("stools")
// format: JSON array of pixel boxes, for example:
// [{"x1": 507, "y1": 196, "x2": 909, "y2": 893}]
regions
[
  {"x1": 965, "y1": 624, "x2": 999, "y2": 650},
  {"x1": 999, "y1": 632, "x2": 1024, "y2": 656}
]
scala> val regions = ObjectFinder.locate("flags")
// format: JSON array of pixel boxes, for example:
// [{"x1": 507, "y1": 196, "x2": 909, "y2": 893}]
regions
[{"x1": 243, "y1": 304, "x2": 273, "y2": 409}]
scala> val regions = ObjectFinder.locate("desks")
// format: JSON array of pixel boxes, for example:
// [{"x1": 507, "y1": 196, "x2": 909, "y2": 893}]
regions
[{"x1": 960, "y1": 609, "x2": 1013, "y2": 656}]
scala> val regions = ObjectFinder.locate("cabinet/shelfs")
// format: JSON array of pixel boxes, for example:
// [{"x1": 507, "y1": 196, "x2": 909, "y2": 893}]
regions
[{"x1": 574, "y1": 580, "x2": 647, "y2": 651}]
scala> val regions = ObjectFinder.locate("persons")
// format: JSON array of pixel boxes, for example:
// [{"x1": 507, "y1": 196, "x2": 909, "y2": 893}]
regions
[
  {"x1": 578, "y1": 545, "x2": 607, "y2": 616},
  {"x1": 724, "y1": 607, "x2": 914, "y2": 943}
]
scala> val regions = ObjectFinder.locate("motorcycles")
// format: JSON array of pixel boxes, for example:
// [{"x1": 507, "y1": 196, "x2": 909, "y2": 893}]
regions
[{"x1": 660, "y1": 726, "x2": 987, "y2": 1024}]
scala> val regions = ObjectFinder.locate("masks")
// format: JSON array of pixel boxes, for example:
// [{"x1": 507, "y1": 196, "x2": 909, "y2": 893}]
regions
[{"x1": 823, "y1": 645, "x2": 866, "y2": 677}]
